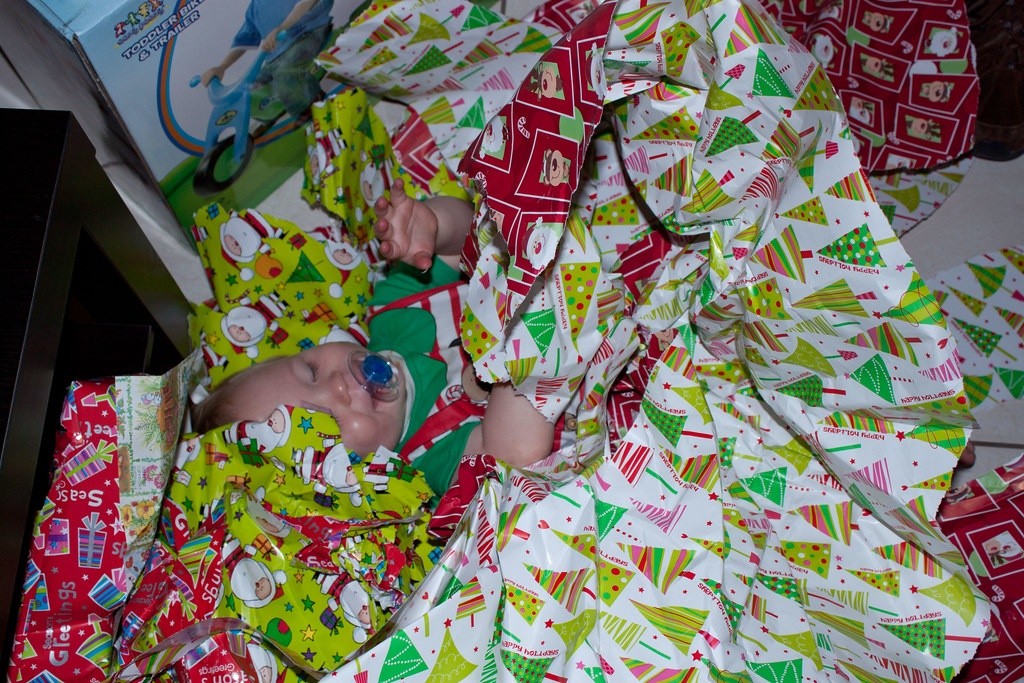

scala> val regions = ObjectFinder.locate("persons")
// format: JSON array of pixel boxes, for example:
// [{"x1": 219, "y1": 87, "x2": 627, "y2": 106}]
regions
[
  {"x1": 201, "y1": 0, "x2": 335, "y2": 119},
  {"x1": 193, "y1": 177, "x2": 975, "y2": 496}
]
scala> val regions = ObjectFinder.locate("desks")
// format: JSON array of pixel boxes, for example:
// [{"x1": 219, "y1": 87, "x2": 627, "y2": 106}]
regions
[{"x1": 0, "y1": 110, "x2": 195, "y2": 683}]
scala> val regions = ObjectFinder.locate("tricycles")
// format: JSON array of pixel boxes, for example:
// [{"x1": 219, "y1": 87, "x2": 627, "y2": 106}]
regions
[{"x1": 186, "y1": 28, "x2": 339, "y2": 195}]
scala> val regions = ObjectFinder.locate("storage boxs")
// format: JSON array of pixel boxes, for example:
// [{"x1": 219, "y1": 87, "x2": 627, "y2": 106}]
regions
[{"x1": 9, "y1": 0, "x2": 371, "y2": 258}]
